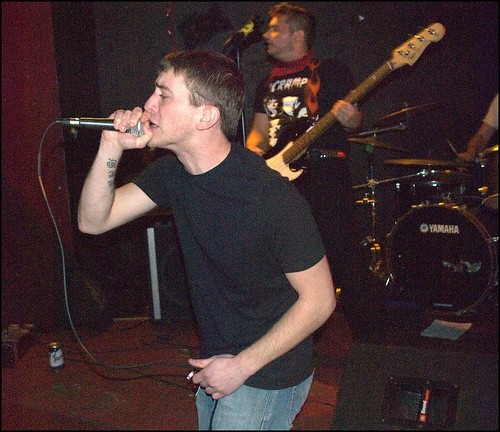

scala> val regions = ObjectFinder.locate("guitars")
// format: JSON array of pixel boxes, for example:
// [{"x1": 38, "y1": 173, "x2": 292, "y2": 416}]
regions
[{"x1": 260, "y1": 23, "x2": 446, "y2": 180}]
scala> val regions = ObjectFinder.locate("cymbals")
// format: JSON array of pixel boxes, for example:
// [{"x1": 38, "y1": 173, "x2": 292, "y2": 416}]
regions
[
  {"x1": 382, "y1": 158, "x2": 463, "y2": 170},
  {"x1": 373, "y1": 99, "x2": 439, "y2": 127},
  {"x1": 347, "y1": 133, "x2": 412, "y2": 156}
]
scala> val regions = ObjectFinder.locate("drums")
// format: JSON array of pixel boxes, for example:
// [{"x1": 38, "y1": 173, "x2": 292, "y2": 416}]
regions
[
  {"x1": 480, "y1": 144, "x2": 499, "y2": 209},
  {"x1": 385, "y1": 202, "x2": 498, "y2": 318}
]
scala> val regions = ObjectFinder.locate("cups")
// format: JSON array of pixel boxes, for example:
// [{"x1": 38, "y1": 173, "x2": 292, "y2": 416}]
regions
[{"x1": 48, "y1": 342, "x2": 65, "y2": 370}]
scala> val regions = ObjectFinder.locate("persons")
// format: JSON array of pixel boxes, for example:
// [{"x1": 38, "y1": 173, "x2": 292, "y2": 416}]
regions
[
  {"x1": 76, "y1": 2, "x2": 362, "y2": 431},
  {"x1": 456, "y1": 91, "x2": 498, "y2": 162}
]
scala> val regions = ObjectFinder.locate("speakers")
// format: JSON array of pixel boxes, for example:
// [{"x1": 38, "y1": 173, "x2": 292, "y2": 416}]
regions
[{"x1": 330, "y1": 342, "x2": 500, "y2": 432}]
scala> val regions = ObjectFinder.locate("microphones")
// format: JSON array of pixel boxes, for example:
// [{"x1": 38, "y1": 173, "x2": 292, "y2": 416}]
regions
[
  {"x1": 55, "y1": 118, "x2": 147, "y2": 138},
  {"x1": 223, "y1": 14, "x2": 262, "y2": 49}
]
